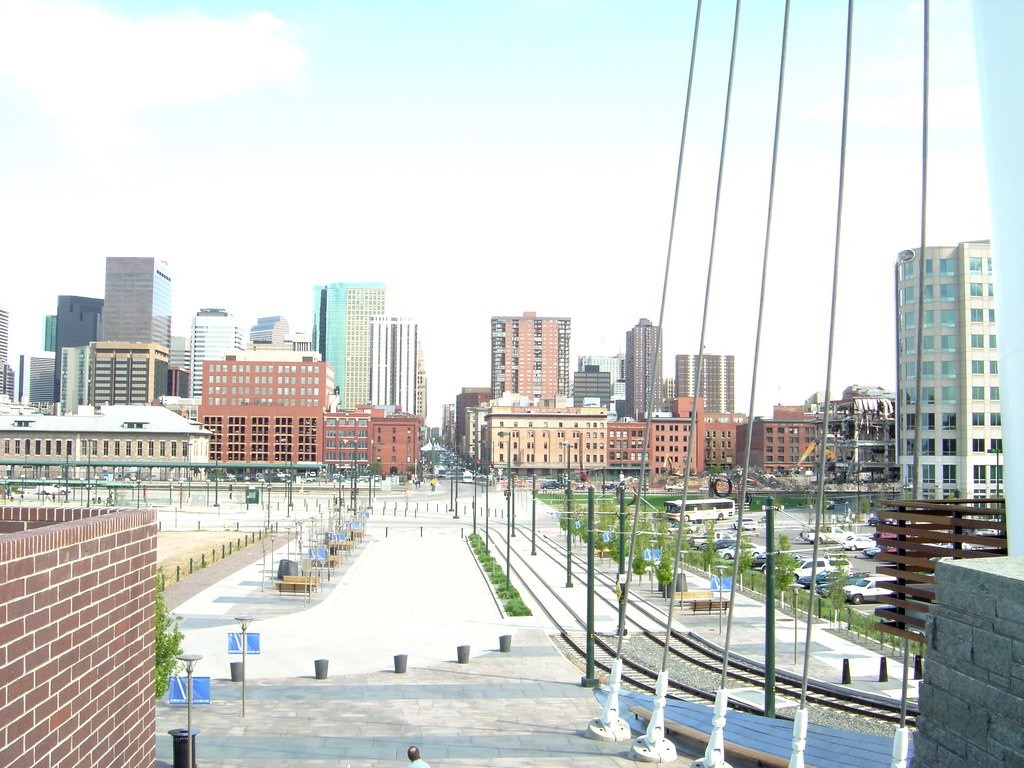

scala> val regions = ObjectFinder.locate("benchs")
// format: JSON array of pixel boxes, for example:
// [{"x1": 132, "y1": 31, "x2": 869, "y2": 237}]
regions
[
  {"x1": 343, "y1": 529, "x2": 362, "y2": 538},
  {"x1": 597, "y1": 551, "x2": 612, "y2": 564},
  {"x1": 689, "y1": 599, "x2": 730, "y2": 615},
  {"x1": 327, "y1": 540, "x2": 354, "y2": 550},
  {"x1": 312, "y1": 555, "x2": 341, "y2": 568},
  {"x1": 676, "y1": 592, "x2": 714, "y2": 603},
  {"x1": 274, "y1": 575, "x2": 320, "y2": 594}
]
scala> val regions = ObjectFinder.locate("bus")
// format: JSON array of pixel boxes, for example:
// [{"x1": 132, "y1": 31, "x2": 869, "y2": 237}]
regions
[
  {"x1": 452, "y1": 469, "x2": 474, "y2": 484},
  {"x1": 433, "y1": 464, "x2": 446, "y2": 478},
  {"x1": 664, "y1": 498, "x2": 734, "y2": 521}
]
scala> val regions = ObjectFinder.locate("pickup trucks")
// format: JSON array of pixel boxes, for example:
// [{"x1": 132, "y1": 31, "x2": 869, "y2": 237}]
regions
[
  {"x1": 36, "y1": 484, "x2": 72, "y2": 495},
  {"x1": 790, "y1": 557, "x2": 853, "y2": 581},
  {"x1": 541, "y1": 480, "x2": 568, "y2": 489},
  {"x1": 665, "y1": 484, "x2": 689, "y2": 491},
  {"x1": 803, "y1": 526, "x2": 845, "y2": 544}
]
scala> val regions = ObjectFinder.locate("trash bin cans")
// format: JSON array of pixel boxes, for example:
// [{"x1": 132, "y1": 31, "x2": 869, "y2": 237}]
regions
[
  {"x1": 168, "y1": 729, "x2": 200, "y2": 768},
  {"x1": 245, "y1": 486, "x2": 259, "y2": 503}
]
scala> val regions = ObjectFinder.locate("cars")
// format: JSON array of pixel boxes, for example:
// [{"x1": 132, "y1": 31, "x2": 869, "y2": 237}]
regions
[
  {"x1": 732, "y1": 517, "x2": 758, "y2": 531},
  {"x1": 839, "y1": 534, "x2": 881, "y2": 558},
  {"x1": 225, "y1": 471, "x2": 380, "y2": 481},
  {"x1": 800, "y1": 527, "x2": 827, "y2": 536},
  {"x1": 868, "y1": 515, "x2": 893, "y2": 525},
  {"x1": 667, "y1": 522, "x2": 873, "y2": 597},
  {"x1": 699, "y1": 486, "x2": 710, "y2": 492}
]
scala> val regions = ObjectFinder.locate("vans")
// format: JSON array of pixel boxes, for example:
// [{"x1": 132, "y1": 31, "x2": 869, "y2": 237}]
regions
[{"x1": 844, "y1": 575, "x2": 897, "y2": 604}]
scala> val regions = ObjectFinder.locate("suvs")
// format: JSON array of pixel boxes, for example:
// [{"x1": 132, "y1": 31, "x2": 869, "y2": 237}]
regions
[{"x1": 601, "y1": 483, "x2": 623, "y2": 490}]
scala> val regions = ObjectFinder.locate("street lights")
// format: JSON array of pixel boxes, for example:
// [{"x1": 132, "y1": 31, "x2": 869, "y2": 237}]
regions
[
  {"x1": 470, "y1": 431, "x2": 511, "y2": 590},
  {"x1": 176, "y1": 615, "x2": 254, "y2": 768},
  {"x1": 989, "y1": 450, "x2": 1000, "y2": 549}
]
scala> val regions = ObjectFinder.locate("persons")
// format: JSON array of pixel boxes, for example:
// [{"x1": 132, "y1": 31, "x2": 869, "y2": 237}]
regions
[
  {"x1": 407, "y1": 746, "x2": 431, "y2": 768},
  {"x1": 110, "y1": 492, "x2": 114, "y2": 505},
  {"x1": 52, "y1": 492, "x2": 55, "y2": 502}
]
